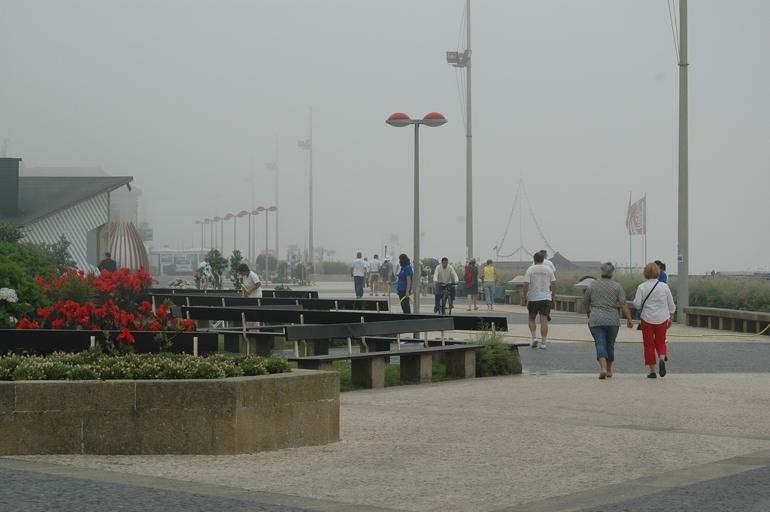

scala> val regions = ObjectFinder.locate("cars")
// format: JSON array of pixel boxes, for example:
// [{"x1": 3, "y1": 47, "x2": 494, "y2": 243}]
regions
[{"x1": 224, "y1": 269, "x2": 298, "y2": 283}]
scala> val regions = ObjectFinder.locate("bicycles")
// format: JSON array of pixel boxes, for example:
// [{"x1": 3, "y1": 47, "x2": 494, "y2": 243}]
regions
[{"x1": 438, "y1": 282, "x2": 459, "y2": 316}]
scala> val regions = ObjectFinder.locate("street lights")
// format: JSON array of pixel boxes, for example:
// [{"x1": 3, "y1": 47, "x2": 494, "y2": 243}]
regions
[
  {"x1": 446, "y1": 49, "x2": 477, "y2": 281},
  {"x1": 195, "y1": 205, "x2": 280, "y2": 288},
  {"x1": 383, "y1": 111, "x2": 449, "y2": 343},
  {"x1": 266, "y1": 162, "x2": 279, "y2": 236},
  {"x1": 298, "y1": 137, "x2": 315, "y2": 268}
]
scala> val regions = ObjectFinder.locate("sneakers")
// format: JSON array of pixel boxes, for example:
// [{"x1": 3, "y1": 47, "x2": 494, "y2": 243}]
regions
[
  {"x1": 532, "y1": 339, "x2": 538, "y2": 348},
  {"x1": 607, "y1": 372, "x2": 613, "y2": 377},
  {"x1": 659, "y1": 359, "x2": 666, "y2": 377},
  {"x1": 539, "y1": 344, "x2": 546, "y2": 349},
  {"x1": 599, "y1": 368, "x2": 607, "y2": 379},
  {"x1": 647, "y1": 373, "x2": 656, "y2": 378}
]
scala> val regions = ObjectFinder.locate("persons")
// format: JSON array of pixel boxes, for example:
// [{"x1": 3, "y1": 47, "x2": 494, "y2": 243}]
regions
[
  {"x1": 654, "y1": 261, "x2": 667, "y2": 283},
  {"x1": 350, "y1": 251, "x2": 428, "y2": 313},
  {"x1": 633, "y1": 262, "x2": 677, "y2": 378},
  {"x1": 521, "y1": 251, "x2": 557, "y2": 349},
  {"x1": 480, "y1": 260, "x2": 496, "y2": 311},
  {"x1": 465, "y1": 257, "x2": 479, "y2": 311},
  {"x1": 582, "y1": 262, "x2": 633, "y2": 379},
  {"x1": 98, "y1": 253, "x2": 117, "y2": 273},
  {"x1": 432, "y1": 258, "x2": 459, "y2": 313},
  {"x1": 238, "y1": 263, "x2": 263, "y2": 299},
  {"x1": 533, "y1": 249, "x2": 556, "y2": 321}
]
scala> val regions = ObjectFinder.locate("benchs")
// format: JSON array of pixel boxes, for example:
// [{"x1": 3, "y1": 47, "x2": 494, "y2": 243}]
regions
[{"x1": 0, "y1": 282, "x2": 534, "y2": 391}]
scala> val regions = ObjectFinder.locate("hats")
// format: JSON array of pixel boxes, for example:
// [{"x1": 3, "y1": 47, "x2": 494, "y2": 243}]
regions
[{"x1": 385, "y1": 256, "x2": 392, "y2": 260}]
